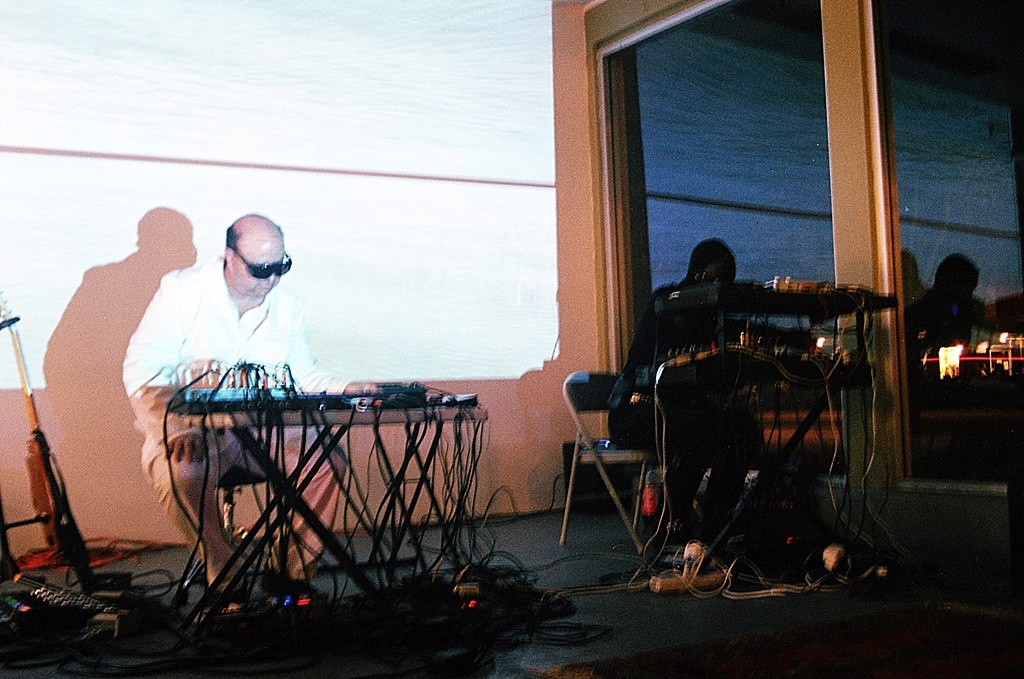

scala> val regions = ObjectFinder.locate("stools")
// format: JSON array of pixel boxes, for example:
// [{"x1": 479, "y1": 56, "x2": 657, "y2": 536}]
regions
[{"x1": 182, "y1": 466, "x2": 266, "y2": 590}]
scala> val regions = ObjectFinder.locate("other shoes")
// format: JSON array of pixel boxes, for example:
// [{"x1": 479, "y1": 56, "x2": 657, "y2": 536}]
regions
[
  {"x1": 260, "y1": 547, "x2": 329, "y2": 602},
  {"x1": 205, "y1": 556, "x2": 248, "y2": 614},
  {"x1": 657, "y1": 519, "x2": 688, "y2": 568}
]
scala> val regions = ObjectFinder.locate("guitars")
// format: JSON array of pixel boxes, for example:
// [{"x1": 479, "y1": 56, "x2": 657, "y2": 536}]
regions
[{"x1": 1, "y1": 291, "x2": 88, "y2": 573}]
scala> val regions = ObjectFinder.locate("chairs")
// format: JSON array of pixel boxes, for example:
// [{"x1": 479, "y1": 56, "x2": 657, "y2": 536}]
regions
[{"x1": 557, "y1": 369, "x2": 654, "y2": 555}]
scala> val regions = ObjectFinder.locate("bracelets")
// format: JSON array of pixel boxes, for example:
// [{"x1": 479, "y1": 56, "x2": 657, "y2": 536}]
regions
[{"x1": 363, "y1": 385, "x2": 370, "y2": 393}]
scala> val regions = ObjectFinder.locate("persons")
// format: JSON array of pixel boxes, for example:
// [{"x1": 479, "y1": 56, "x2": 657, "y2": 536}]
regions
[
  {"x1": 122, "y1": 216, "x2": 378, "y2": 609},
  {"x1": 905, "y1": 254, "x2": 987, "y2": 361},
  {"x1": 605, "y1": 240, "x2": 765, "y2": 548}
]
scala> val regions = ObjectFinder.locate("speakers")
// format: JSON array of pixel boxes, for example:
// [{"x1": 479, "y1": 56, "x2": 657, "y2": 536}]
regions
[{"x1": 561, "y1": 439, "x2": 641, "y2": 517}]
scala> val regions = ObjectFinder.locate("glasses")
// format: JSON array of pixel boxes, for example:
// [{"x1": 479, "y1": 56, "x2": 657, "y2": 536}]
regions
[{"x1": 227, "y1": 245, "x2": 292, "y2": 279}]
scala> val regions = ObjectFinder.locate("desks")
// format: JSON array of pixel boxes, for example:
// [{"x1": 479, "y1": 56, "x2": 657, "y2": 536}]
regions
[{"x1": 177, "y1": 408, "x2": 488, "y2": 607}]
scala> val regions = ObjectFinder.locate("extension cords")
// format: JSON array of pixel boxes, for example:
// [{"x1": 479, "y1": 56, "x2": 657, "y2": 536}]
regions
[{"x1": 649, "y1": 573, "x2": 726, "y2": 592}]
